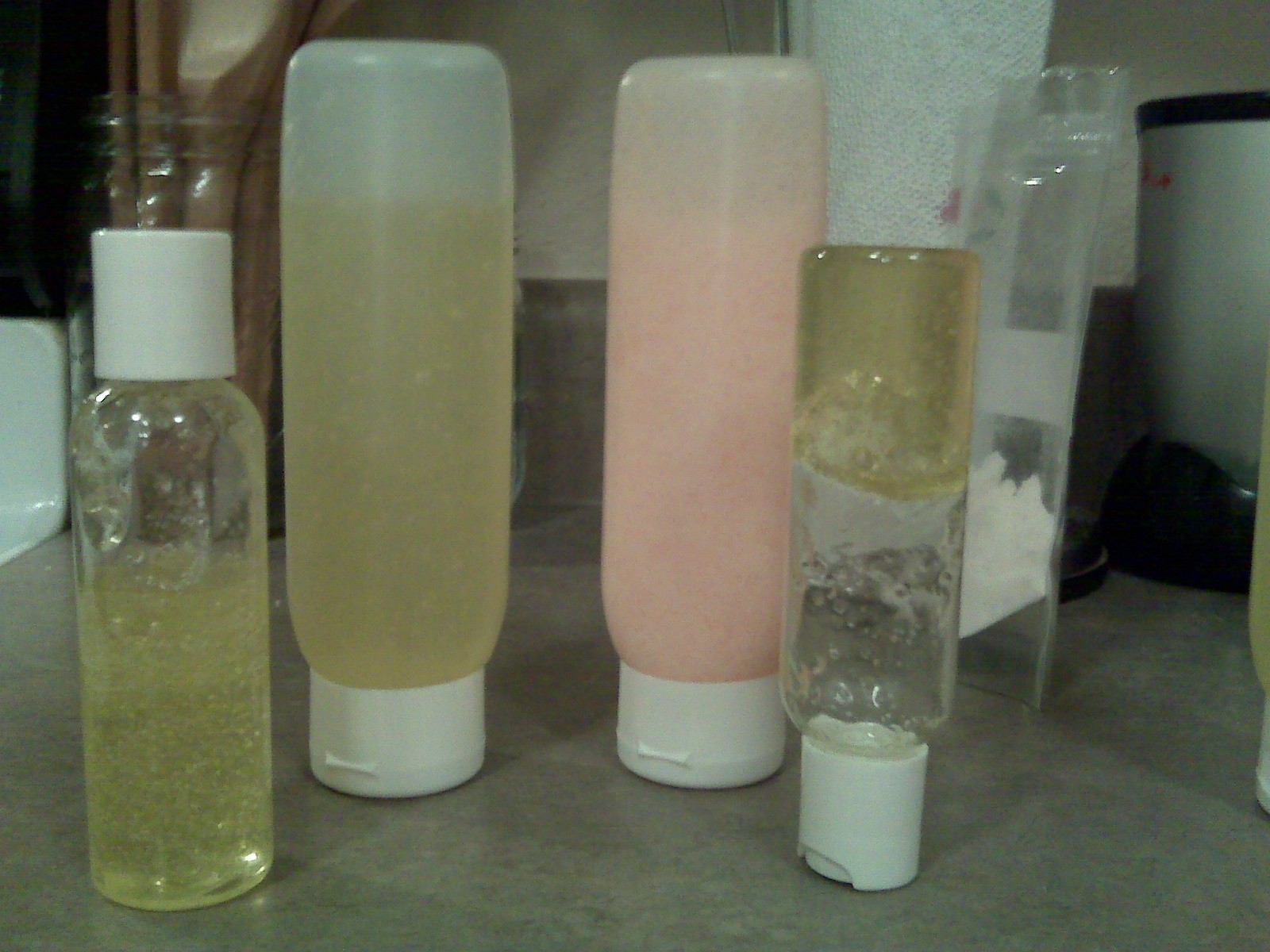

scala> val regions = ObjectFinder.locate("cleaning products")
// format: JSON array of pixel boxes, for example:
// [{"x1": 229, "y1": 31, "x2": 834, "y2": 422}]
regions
[{"x1": 281, "y1": 37, "x2": 515, "y2": 802}]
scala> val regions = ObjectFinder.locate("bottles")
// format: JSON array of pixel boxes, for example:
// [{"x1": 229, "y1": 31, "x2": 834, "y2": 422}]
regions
[
  {"x1": 600, "y1": 56, "x2": 825, "y2": 788},
  {"x1": 1249, "y1": 386, "x2": 1270, "y2": 817},
  {"x1": 782, "y1": 244, "x2": 975, "y2": 891},
  {"x1": 74, "y1": 230, "x2": 275, "y2": 907},
  {"x1": 279, "y1": 39, "x2": 515, "y2": 796}
]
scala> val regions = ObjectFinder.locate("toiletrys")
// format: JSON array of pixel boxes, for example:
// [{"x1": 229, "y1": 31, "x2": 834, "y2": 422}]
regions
[
  {"x1": 776, "y1": 242, "x2": 986, "y2": 896},
  {"x1": 597, "y1": 52, "x2": 832, "y2": 792},
  {"x1": 64, "y1": 226, "x2": 273, "y2": 913}
]
